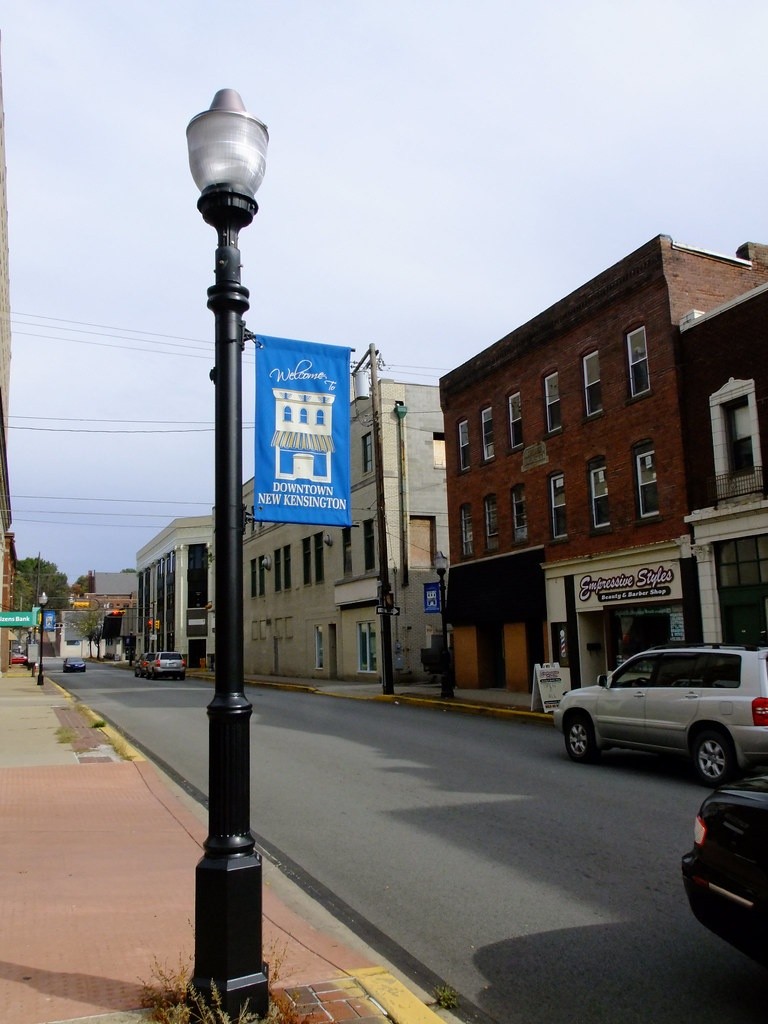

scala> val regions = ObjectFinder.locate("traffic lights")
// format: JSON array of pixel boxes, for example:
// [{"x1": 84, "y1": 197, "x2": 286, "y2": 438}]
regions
[
  {"x1": 113, "y1": 610, "x2": 126, "y2": 616},
  {"x1": 155, "y1": 620, "x2": 160, "y2": 630}
]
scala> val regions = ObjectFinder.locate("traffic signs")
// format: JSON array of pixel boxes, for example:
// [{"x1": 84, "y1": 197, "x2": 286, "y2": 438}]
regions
[{"x1": 375, "y1": 606, "x2": 401, "y2": 616}]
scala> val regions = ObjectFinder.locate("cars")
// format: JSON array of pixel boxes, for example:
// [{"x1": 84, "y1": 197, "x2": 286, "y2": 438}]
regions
[
  {"x1": 62, "y1": 657, "x2": 87, "y2": 673},
  {"x1": 683, "y1": 767, "x2": 767, "y2": 964},
  {"x1": 9, "y1": 652, "x2": 27, "y2": 663}
]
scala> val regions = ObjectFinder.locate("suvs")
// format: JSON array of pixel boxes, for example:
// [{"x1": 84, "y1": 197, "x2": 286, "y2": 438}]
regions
[
  {"x1": 551, "y1": 643, "x2": 768, "y2": 787},
  {"x1": 134, "y1": 652, "x2": 156, "y2": 678},
  {"x1": 145, "y1": 650, "x2": 188, "y2": 680}
]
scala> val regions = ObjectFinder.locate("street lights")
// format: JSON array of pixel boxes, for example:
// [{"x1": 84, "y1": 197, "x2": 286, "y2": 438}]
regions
[
  {"x1": 432, "y1": 550, "x2": 455, "y2": 700},
  {"x1": 38, "y1": 592, "x2": 48, "y2": 685},
  {"x1": 185, "y1": 85, "x2": 267, "y2": 1024}
]
